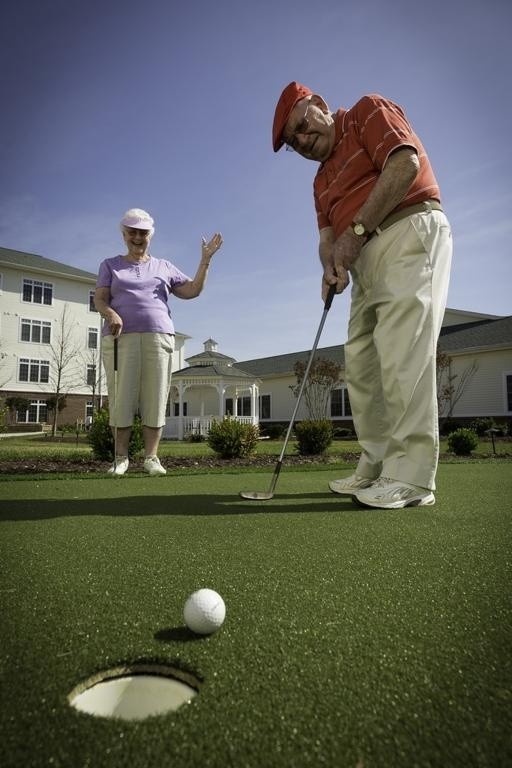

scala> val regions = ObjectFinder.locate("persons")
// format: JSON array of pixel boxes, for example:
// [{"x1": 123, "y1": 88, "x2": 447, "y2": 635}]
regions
[
  {"x1": 94, "y1": 207, "x2": 224, "y2": 477},
  {"x1": 272, "y1": 80, "x2": 454, "y2": 509}
]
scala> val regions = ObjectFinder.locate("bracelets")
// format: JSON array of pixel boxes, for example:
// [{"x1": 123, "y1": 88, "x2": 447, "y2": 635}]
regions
[{"x1": 199, "y1": 262, "x2": 210, "y2": 265}]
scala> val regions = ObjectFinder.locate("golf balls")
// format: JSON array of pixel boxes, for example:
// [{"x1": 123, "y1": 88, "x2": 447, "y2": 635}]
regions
[{"x1": 183, "y1": 589, "x2": 226, "y2": 634}]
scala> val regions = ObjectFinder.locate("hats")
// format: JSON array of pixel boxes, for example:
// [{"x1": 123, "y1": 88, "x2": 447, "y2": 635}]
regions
[
  {"x1": 272, "y1": 82, "x2": 313, "y2": 154},
  {"x1": 120, "y1": 208, "x2": 153, "y2": 231}
]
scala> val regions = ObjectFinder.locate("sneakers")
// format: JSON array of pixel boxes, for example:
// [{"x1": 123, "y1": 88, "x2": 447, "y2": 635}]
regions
[
  {"x1": 351, "y1": 477, "x2": 436, "y2": 510},
  {"x1": 144, "y1": 456, "x2": 167, "y2": 478},
  {"x1": 106, "y1": 455, "x2": 131, "y2": 476},
  {"x1": 328, "y1": 473, "x2": 375, "y2": 496}
]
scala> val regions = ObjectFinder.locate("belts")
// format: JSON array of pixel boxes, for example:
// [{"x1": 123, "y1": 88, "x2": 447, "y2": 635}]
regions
[{"x1": 362, "y1": 198, "x2": 447, "y2": 244}]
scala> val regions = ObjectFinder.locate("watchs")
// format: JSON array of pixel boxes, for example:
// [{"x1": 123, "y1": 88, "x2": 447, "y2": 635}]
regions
[{"x1": 350, "y1": 220, "x2": 372, "y2": 240}]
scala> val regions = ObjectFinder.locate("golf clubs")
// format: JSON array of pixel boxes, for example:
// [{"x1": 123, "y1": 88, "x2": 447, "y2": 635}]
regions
[
  {"x1": 239, "y1": 285, "x2": 338, "y2": 499},
  {"x1": 112, "y1": 338, "x2": 119, "y2": 474}
]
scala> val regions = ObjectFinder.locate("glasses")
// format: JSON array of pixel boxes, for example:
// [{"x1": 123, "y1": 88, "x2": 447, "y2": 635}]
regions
[
  {"x1": 124, "y1": 229, "x2": 149, "y2": 238},
  {"x1": 285, "y1": 99, "x2": 311, "y2": 152}
]
